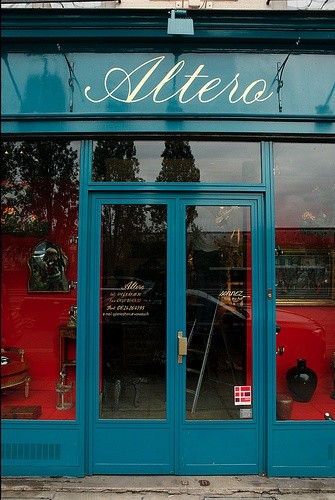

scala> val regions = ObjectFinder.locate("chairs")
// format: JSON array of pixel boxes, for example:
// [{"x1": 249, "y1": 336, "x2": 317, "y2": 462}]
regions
[{"x1": 0, "y1": 344, "x2": 32, "y2": 401}]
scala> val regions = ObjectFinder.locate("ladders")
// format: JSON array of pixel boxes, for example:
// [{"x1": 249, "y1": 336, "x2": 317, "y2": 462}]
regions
[{"x1": 183, "y1": 287, "x2": 238, "y2": 415}]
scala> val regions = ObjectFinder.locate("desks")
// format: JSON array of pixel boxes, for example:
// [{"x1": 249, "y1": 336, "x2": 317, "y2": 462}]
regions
[{"x1": 55, "y1": 322, "x2": 78, "y2": 411}]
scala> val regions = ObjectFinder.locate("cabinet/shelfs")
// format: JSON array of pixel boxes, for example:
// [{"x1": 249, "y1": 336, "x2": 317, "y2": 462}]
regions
[{"x1": 275, "y1": 243, "x2": 334, "y2": 301}]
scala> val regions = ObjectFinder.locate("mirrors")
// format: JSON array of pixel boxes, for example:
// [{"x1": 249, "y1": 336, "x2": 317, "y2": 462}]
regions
[{"x1": 26, "y1": 237, "x2": 72, "y2": 294}]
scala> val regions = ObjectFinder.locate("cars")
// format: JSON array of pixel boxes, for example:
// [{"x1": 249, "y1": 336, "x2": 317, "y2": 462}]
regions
[{"x1": 98, "y1": 275, "x2": 247, "y2": 347}]
